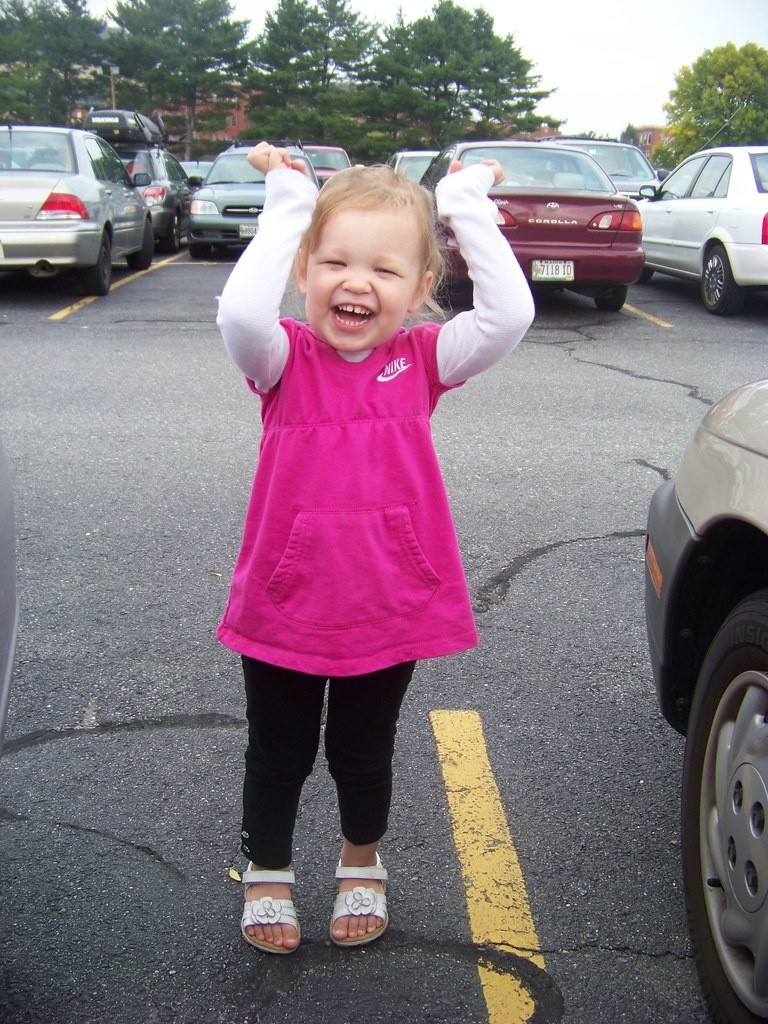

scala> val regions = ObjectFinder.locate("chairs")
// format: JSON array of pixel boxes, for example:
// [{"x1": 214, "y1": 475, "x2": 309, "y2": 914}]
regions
[
  {"x1": 554, "y1": 173, "x2": 587, "y2": 188},
  {"x1": 28, "y1": 149, "x2": 67, "y2": 170}
]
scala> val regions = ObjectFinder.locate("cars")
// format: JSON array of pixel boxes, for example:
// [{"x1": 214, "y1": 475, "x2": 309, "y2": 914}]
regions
[
  {"x1": 385, "y1": 150, "x2": 438, "y2": 186},
  {"x1": 176, "y1": 160, "x2": 216, "y2": 188},
  {"x1": 642, "y1": 375, "x2": 768, "y2": 1024},
  {"x1": 415, "y1": 140, "x2": 646, "y2": 314},
  {"x1": 290, "y1": 144, "x2": 364, "y2": 191},
  {"x1": 629, "y1": 144, "x2": 768, "y2": 318}
]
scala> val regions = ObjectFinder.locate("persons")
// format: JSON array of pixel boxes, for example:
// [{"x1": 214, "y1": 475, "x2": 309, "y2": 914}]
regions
[{"x1": 218, "y1": 141, "x2": 535, "y2": 955}]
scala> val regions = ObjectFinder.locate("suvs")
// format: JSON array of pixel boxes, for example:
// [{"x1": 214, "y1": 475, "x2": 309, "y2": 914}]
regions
[
  {"x1": 1, "y1": 125, "x2": 157, "y2": 298},
  {"x1": 534, "y1": 134, "x2": 673, "y2": 203},
  {"x1": 184, "y1": 140, "x2": 328, "y2": 261},
  {"x1": 81, "y1": 106, "x2": 197, "y2": 257}
]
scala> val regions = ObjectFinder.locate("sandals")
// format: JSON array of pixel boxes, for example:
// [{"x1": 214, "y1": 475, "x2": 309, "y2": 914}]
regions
[
  {"x1": 330, "y1": 852, "x2": 388, "y2": 947},
  {"x1": 241, "y1": 861, "x2": 301, "y2": 954}
]
